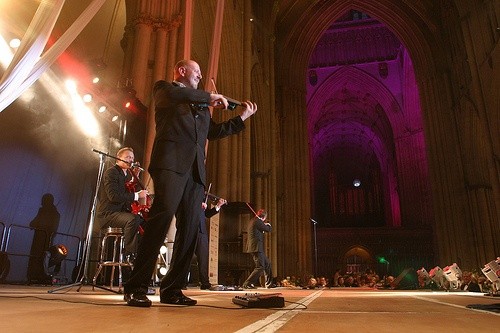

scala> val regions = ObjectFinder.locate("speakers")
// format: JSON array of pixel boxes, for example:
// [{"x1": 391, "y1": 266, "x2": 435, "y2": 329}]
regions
[{"x1": 394, "y1": 268, "x2": 417, "y2": 290}]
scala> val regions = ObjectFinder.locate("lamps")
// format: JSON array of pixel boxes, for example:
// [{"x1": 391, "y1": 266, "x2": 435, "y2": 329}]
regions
[
  {"x1": 40, "y1": 243, "x2": 68, "y2": 277},
  {"x1": 480, "y1": 257, "x2": 500, "y2": 296},
  {"x1": 442, "y1": 263, "x2": 468, "y2": 291},
  {"x1": 416, "y1": 267, "x2": 429, "y2": 289},
  {"x1": 428, "y1": 266, "x2": 443, "y2": 290}
]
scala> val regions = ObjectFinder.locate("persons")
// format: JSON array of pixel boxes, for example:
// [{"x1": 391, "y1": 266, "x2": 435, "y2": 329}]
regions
[
  {"x1": 123, "y1": 59, "x2": 258, "y2": 307},
  {"x1": 242, "y1": 209, "x2": 277, "y2": 289},
  {"x1": 273, "y1": 270, "x2": 496, "y2": 295},
  {"x1": 93, "y1": 147, "x2": 150, "y2": 266},
  {"x1": 182, "y1": 185, "x2": 228, "y2": 291}
]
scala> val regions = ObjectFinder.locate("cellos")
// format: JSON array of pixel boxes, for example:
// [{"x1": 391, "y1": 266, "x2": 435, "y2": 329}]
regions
[{"x1": 126, "y1": 166, "x2": 169, "y2": 269}]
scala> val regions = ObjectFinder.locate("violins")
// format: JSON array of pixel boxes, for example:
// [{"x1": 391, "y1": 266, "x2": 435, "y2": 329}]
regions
[
  {"x1": 205, "y1": 191, "x2": 227, "y2": 205},
  {"x1": 191, "y1": 94, "x2": 257, "y2": 115}
]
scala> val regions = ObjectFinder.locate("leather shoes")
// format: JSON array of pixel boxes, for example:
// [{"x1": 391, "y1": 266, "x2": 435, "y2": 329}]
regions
[
  {"x1": 126, "y1": 293, "x2": 152, "y2": 307},
  {"x1": 160, "y1": 292, "x2": 197, "y2": 305}
]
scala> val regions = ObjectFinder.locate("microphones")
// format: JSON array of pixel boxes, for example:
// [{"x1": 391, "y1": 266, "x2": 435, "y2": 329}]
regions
[
  {"x1": 310, "y1": 218, "x2": 318, "y2": 223},
  {"x1": 131, "y1": 163, "x2": 144, "y2": 171}
]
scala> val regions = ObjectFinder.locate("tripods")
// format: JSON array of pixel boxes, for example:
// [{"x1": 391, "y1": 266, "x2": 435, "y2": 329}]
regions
[{"x1": 47, "y1": 155, "x2": 122, "y2": 293}]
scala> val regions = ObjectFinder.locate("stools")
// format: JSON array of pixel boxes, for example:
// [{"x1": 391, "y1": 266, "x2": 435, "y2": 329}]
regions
[{"x1": 91, "y1": 227, "x2": 134, "y2": 292}]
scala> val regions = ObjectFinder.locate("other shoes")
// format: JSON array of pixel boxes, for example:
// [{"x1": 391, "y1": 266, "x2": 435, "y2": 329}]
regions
[
  {"x1": 182, "y1": 287, "x2": 187, "y2": 290},
  {"x1": 200, "y1": 285, "x2": 216, "y2": 291},
  {"x1": 267, "y1": 285, "x2": 276, "y2": 288},
  {"x1": 122, "y1": 253, "x2": 136, "y2": 263},
  {"x1": 242, "y1": 285, "x2": 254, "y2": 289}
]
